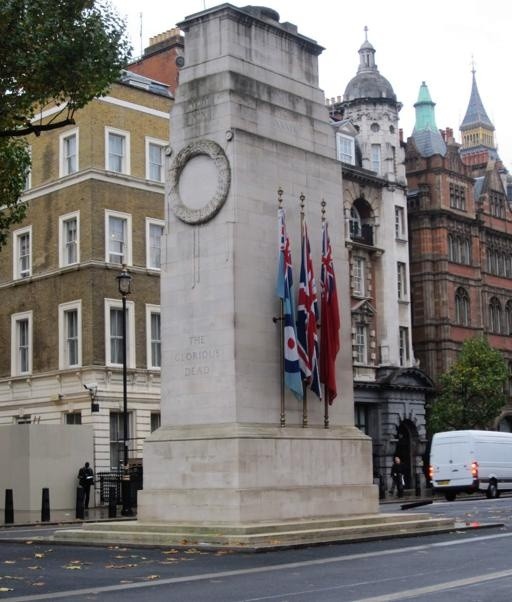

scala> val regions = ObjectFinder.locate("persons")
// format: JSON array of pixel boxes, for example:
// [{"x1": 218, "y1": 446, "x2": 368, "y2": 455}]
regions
[
  {"x1": 77, "y1": 462, "x2": 95, "y2": 509},
  {"x1": 388, "y1": 457, "x2": 408, "y2": 498}
]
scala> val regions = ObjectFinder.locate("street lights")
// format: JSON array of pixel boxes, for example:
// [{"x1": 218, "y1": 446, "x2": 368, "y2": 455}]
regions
[{"x1": 115, "y1": 265, "x2": 134, "y2": 517}]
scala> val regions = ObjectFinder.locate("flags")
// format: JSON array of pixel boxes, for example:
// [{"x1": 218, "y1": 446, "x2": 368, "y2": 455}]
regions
[{"x1": 276, "y1": 210, "x2": 339, "y2": 406}]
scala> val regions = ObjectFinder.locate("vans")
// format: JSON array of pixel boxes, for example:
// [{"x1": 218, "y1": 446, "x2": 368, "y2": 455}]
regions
[{"x1": 429, "y1": 430, "x2": 512, "y2": 501}]
